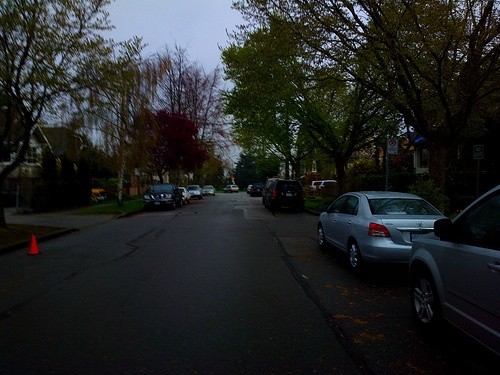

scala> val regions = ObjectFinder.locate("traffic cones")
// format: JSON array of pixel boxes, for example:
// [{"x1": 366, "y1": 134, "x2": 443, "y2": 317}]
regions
[{"x1": 27, "y1": 235, "x2": 39, "y2": 254}]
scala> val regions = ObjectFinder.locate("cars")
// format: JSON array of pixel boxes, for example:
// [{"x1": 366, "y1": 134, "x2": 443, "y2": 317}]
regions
[
  {"x1": 261, "y1": 177, "x2": 306, "y2": 212},
  {"x1": 247, "y1": 183, "x2": 265, "y2": 196},
  {"x1": 311, "y1": 180, "x2": 337, "y2": 191},
  {"x1": 410, "y1": 183, "x2": 500, "y2": 364},
  {"x1": 143, "y1": 183, "x2": 189, "y2": 211},
  {"x1": 223, "y1": 185, "x2": 239, "y2": 193},
  {"x1": 187, "y1": 184, "x2": 203, "y2": 200},
  {"x1": 202, "y1": 185, "x2": 216, "y2": 196},
  {"x1": 317, "y1": 191, "x2": 455, "y2": 273}
]
